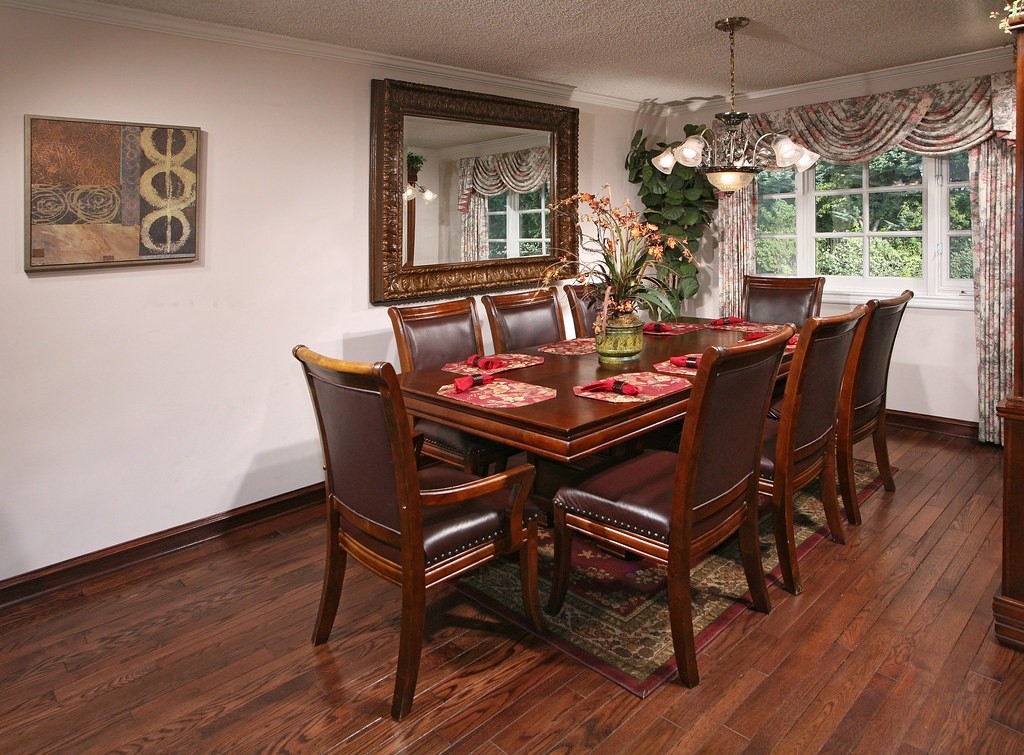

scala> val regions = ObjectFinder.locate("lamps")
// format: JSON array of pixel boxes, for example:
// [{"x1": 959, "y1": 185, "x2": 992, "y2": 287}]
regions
[
  {"x1": 404, "y1": 181, "x2": 438, "y2": 205},
  {"x1": 650, "y1": 18, "x2": 821, "y2": 199}
]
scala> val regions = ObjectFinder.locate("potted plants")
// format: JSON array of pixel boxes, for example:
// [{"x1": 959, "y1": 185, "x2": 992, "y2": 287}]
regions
[{"x1": 405, "y1": 151, "x2": 427, "y2": 182}]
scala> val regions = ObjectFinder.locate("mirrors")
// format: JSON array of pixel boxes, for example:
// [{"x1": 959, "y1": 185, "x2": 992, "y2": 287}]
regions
[{"x1": 372, "y1": 77, "x2": 580, "y2": 304}]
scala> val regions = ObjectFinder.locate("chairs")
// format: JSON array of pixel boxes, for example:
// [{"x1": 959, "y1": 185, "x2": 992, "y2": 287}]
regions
[
  {"x1": 770, "y1": 290, "x2": 915, "y2": 526},
  {"x1": 290, "y1": 342, "x2": 546, "y2": 722},
  {"x1": 480, "y1": 285, "x2": 566, "y2": 354},
  {"x1": 756, "y1": 304, "x2": 870, "y2": 595},
  {"x1": 546, "y1": 321, "x2": 798, "y2": 689},
  {"x1": 742, "y1": 274, "x2": 826, "y2": 329},
  {"x1": 386, "y1": 294, "x2": 529, "y2": 476},
  {"x1": 562, "y1": 280, "x2": 614, "y2": 339}
]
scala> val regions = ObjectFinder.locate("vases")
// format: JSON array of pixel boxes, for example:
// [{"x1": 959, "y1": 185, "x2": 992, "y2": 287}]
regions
[{"x1": 590, "y1": 307, "x2": 646, "y2": 364}]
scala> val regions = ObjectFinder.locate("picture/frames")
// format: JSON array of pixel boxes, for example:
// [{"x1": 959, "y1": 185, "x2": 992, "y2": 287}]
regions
[{"x1": 24, "y1": 113, "x2": 202, "y2": 272}]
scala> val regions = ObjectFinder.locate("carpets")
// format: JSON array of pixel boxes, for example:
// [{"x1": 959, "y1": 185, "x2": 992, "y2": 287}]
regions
[{"x1": 442, "y1": 457, "x2": 899, "y2": 700}]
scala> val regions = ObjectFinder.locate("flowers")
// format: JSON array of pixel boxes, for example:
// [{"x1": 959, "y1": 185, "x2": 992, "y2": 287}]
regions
[{"x1": 526, "y1": 183, "x2": 703, "y2": 335}]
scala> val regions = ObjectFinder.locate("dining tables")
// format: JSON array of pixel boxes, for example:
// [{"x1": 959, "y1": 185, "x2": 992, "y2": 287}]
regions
[{"x1": 392, "y1": 319, "x2": 802, "y2": 522}]
